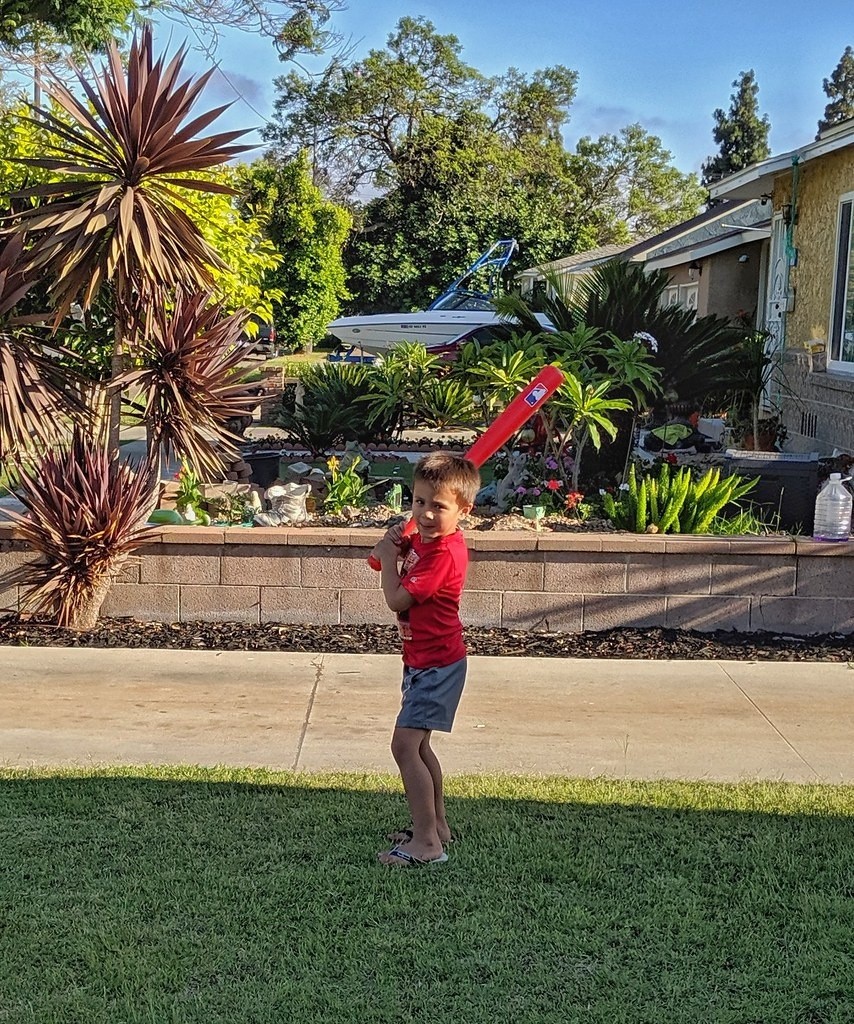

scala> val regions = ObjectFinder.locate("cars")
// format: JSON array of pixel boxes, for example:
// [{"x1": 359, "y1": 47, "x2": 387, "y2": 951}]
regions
[
  {"x1": 251, "y1": 326, "x2": 280, "y2": 344},
  {"x1": 425, "y1": 323, "x2": 560, "y2": 381}
]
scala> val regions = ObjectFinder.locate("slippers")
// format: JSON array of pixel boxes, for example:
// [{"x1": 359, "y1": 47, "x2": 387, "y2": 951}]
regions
[
  {"x1": 389, "y1": 827, "x2": 455, "y2": 845},
  {"x1": 378, "y1": 850, "x2": 448, "y2": 868}
]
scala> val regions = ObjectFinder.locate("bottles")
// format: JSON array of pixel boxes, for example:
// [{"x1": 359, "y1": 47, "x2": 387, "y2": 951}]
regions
[{"x1": 814, "y1": 473, "x2": 852, "y2": 541}]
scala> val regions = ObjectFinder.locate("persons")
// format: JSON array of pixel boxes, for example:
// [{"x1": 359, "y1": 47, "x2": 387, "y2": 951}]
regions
[{"x1": 371, "y1": 451, "x2": 481, "y2": 868}]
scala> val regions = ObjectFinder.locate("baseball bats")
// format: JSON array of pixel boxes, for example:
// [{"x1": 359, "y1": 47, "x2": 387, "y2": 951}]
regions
[{"x1": 367, "y1": 365, "x2": 565, "y2": 572}]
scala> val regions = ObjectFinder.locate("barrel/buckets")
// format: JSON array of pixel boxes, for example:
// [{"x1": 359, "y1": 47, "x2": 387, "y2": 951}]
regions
[
  {"x1": 523, "y1": 505, "x2": 546, "y2": 519},
  {"x1": 243, "y1": 452, "x2": 281, "y2": 488}
]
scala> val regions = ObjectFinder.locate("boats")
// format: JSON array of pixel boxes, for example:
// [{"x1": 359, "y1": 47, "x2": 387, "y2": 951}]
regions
[{"x1": 326, "y1": 240, "x2": 559, "y2": 358}]
